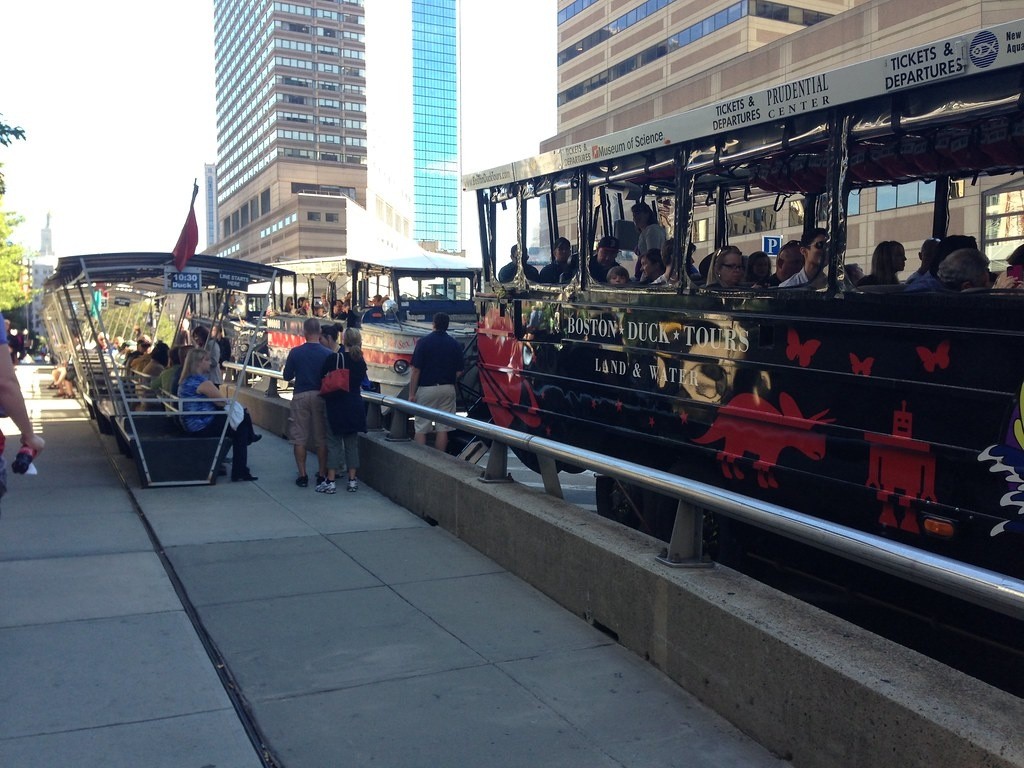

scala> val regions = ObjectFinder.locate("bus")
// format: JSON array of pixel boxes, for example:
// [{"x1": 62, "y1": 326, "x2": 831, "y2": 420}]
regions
[
  {"x1": 147, "y1": 278, "x2": 308, "y2": 371},
  {"x1": 460, "y1": 17, "x2": 1024, "y2": 697},
  {"x1": 261, "y1": 255, "x2": 483, "y2": 431}
]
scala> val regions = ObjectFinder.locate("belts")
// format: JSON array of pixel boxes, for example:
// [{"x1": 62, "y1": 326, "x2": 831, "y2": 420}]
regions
[{"x1": 419, "y1": 383, "x2": 452, "y2": 386}]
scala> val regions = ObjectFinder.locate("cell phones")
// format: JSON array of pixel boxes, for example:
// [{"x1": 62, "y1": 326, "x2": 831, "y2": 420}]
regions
[{"x1": 1006, "y1": 265, "x2": 1022, "y2": 288}]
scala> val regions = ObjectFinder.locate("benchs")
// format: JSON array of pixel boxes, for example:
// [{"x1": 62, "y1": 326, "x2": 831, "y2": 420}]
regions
[{"x1": 75, "y1": 353, "x2": 233, "y2": 488}]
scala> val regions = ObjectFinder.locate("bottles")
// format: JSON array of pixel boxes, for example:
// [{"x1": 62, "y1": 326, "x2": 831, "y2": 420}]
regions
[{"x1": 11, "y1": 444, "x2": 38, "y2": 475}]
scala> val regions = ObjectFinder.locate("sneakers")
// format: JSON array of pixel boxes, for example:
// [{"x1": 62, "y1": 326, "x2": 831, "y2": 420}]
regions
[
  {"x1": 347, "y1": 477, "x2": 359, "y2": 491},
  {"x1": 316, "y1": 479, "x2": 337, "y2": 493}
]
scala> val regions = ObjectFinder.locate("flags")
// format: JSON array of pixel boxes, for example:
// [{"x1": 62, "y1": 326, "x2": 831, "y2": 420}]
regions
[{"x1": 173, "y1": 205, "x2": 198, "y2": 273}]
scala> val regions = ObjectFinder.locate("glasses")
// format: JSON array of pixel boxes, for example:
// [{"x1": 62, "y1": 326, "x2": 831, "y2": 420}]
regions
[
  {"x1": 806, "y1": 241, "x2": 825, "y2": 250},
  {"x1": 602, "y1": 249, "x2": 618, "y2": 258},
  {"x1": 721, "y1": 263, "x2": 744, "y2": 271}
]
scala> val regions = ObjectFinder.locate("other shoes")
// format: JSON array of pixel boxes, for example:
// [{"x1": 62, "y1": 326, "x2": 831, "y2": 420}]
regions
[
  {"x1": 296, "y1": 475, "x2": 308, "y2": 487},
  {"x1": 231, "y1": 473, "x2": 258, "y2": 481},
  {"x1": 247, "y1": 434, "x2": 262, "y2": 445},
  {"x1": 314, "y1": 472, "x2": 325, "y2": 485}
]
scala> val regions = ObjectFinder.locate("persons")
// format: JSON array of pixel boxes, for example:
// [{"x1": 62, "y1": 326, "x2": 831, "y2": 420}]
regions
[
  {"x1": 284, "y1": 291, "x2": 383, "y2": 327},
  {"x1": 46, "y1": 304, "x2": 262, "y2": 482},
  {"x1": 408, "y1": 312, "x2": 465, "y2": 452},
  {"x1": 498, "y1": 202, "x2": 1024, "y2": 293},
  {"x1": 316, "y1": 322, "x2": 371, "y2": 478},
  {"x1": 283, "y1": 318, "x2": 336, "y2": 488},
  {"x1": 0, "y1": 311, "x2": 47, "y2": 502},
  {"x1": 315, "y1": 327, "x2": 368, "y2": 495}
]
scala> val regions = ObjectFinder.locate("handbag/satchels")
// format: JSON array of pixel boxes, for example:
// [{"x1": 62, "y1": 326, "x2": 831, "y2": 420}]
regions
[
  {"x1": 317, "y1": 353, "x2": 349, "y2": 399},
  {"x1": 224, "y1": 400, "x2": 244, "y2": 431}
]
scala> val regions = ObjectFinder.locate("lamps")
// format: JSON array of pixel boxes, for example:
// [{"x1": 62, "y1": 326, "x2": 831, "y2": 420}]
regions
[{"x1": 381, "y1": 300, "x2": 402, "y2": 331}]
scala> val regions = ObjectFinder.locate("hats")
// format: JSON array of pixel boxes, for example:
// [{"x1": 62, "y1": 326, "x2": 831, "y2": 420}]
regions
[
  {"x1": 599, "y1": 237, "x2": 620, "y2": 249},
  {"x1": 937, "y1": 249, "x2": 990, "y2": 286},
  {"x1": 553, "y1": 236, "x2": 570, "y2": 252}
]
